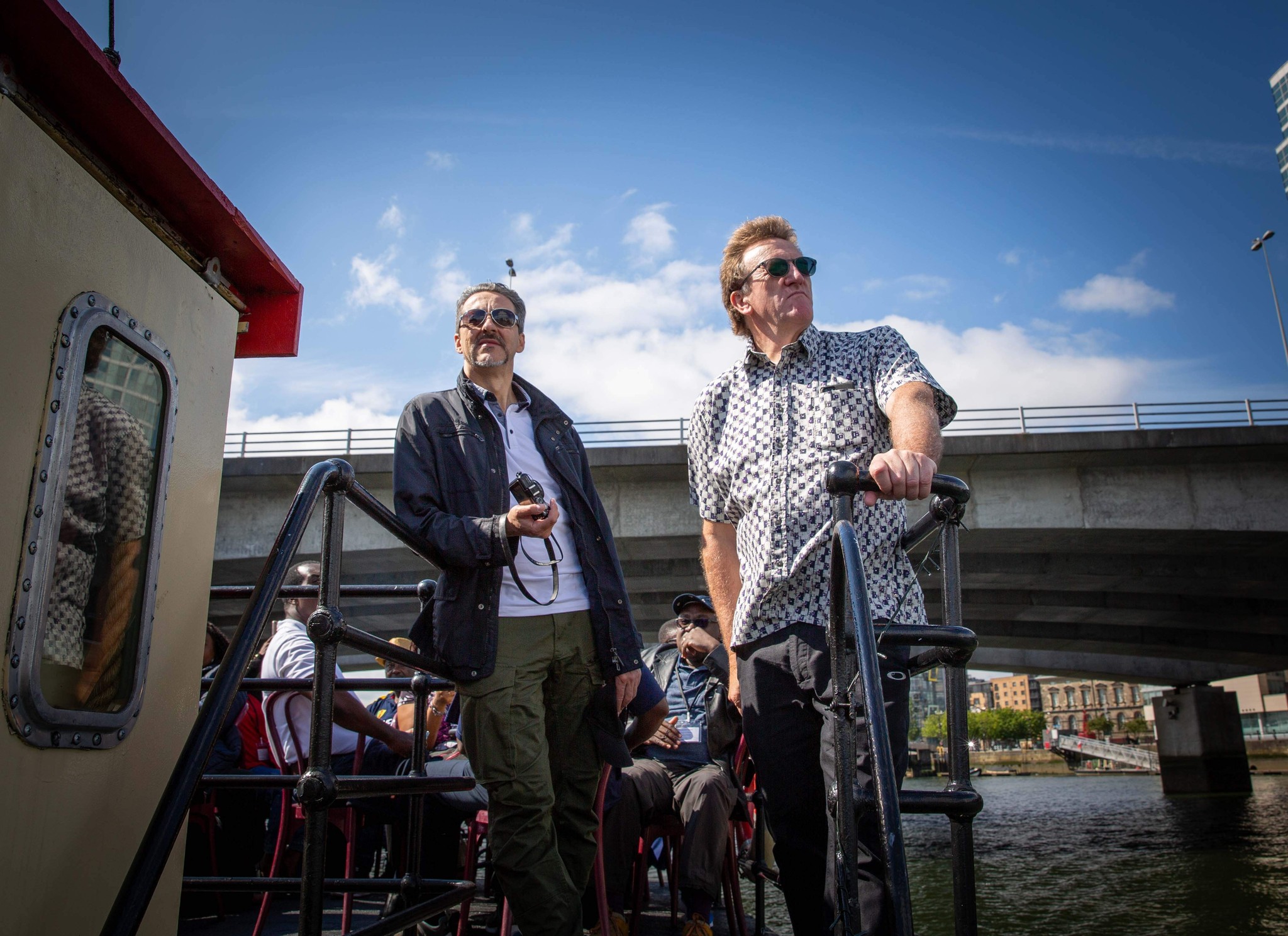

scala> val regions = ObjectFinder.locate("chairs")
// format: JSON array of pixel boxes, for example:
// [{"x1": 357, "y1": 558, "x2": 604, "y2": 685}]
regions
[
  {"x1": 251, "y1": 688, "x2": 367, "y2": 936},
  {"x1": 457, "y1": 762, "x2": 613, "y2": 936},
  {"x1": 631, "y1": 733, "x2": 748, "y2": 936}
]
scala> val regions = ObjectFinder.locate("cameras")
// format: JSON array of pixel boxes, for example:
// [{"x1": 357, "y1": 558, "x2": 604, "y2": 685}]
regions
[{"x1": 509, "y1": 471, "x2": 551, "y2": 521}]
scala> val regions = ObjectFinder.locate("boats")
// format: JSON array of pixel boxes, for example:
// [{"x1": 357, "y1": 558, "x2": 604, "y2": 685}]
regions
[{"x1": 941, "y1": 766, "x2": 982, "y2": 777}]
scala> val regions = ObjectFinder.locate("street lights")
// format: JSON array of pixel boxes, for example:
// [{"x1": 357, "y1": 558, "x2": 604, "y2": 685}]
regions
[{"x1": 1251, "y1": 231, "x2": 1288, "y2": 363}]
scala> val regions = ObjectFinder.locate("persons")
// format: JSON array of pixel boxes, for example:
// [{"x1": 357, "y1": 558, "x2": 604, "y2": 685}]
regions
[
  {"x1": 390, "y1": 281, "x2": 643, "y2": 936},
  {"x1": 687, "y1": 217, "x2": 958, "y2": 936},
  {"x1": 199, "y1": 561, "x2": 744, "y2": 936}
]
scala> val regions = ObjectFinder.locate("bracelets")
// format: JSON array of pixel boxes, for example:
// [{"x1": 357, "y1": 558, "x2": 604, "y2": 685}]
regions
[{"x1": 429, "y1": 702, "x2": 446, "y2": 716}]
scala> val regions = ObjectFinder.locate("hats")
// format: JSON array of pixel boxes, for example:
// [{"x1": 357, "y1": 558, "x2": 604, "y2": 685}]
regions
[
  {"x1": 375, "y1": 637, "x2": 416, "y2": 666},
  {"x1": 672, "y1": 593, "x2": 715, "y2": 616}
]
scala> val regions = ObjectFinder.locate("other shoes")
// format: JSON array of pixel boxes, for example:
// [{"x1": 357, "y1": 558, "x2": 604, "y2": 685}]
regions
[
  {"x1": 590, "y1": 904, "x2": 629, "y2": 936},
  {"x1": 680, "y1": 913, "x2": 713, "y2": 936}
]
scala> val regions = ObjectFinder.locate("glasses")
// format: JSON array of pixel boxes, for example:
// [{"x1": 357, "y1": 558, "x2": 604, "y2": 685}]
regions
[
  {"x1": 675, "y1": 618, "x2": 718, "y2": 628},
  {"x1": 458, "y1": 308, "x2": 520, "y2": 334},
  {"x1": 737, "y1": 256, "x2": 817, "y2": 290}
]
